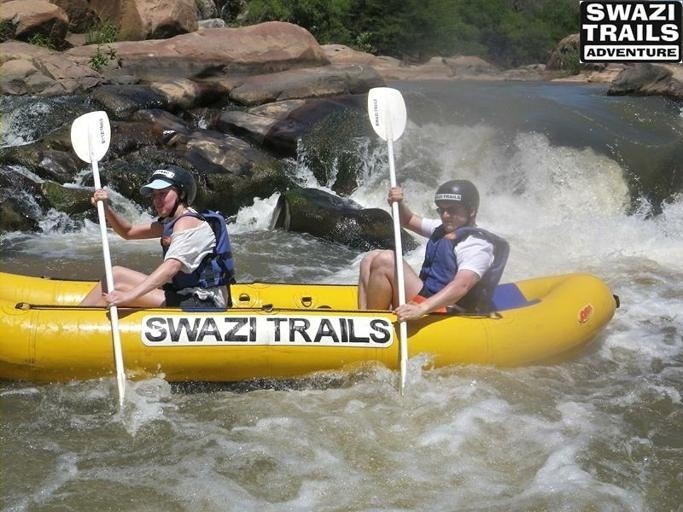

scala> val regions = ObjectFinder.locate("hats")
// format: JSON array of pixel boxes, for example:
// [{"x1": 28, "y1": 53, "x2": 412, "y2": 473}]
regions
[{"x1": 139, "y1": 179, "x2": 174, "y2": 196}]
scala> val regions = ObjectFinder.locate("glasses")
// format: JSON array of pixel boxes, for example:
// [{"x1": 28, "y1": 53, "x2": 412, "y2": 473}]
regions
[{"x1": 436, "y1": 205, "x2": 464, "y2": 213}]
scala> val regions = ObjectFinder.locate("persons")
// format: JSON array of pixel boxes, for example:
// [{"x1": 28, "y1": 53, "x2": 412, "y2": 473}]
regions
[
  {"x1": 76, "y1": 164, "x2": 235, "y2": 312},
  {"x1": 355, "y1": 177, "x2": 512, "y2": 324}
]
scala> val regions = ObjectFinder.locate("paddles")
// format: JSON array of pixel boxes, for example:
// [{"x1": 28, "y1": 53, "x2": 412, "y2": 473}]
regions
[
  {"x1": 71, "y1": 109, "x2": 125, "y2": 409},
  {"x1": 367, "y1": 86, "x2": 407, "y2": 395}
]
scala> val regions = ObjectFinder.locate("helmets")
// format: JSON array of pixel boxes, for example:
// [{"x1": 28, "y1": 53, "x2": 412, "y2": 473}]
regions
[
  {"x1": 435, "y1": 179, "x2": 480, "y2": 209},
  {"x1": 150, "y1": 164, "x2": 197, "y2": 206}
]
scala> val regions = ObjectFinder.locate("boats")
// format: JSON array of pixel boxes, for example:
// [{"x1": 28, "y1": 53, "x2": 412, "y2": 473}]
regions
[{"x1": 0, "y1": 265, "x2": 619, "y2": 385}]
